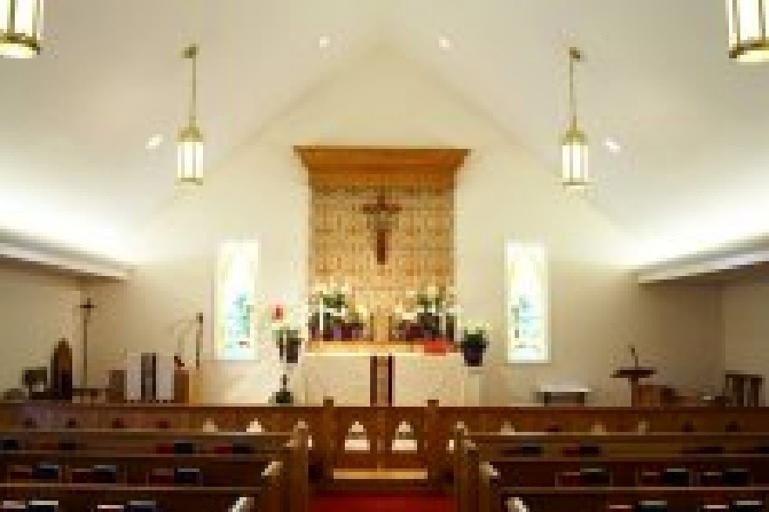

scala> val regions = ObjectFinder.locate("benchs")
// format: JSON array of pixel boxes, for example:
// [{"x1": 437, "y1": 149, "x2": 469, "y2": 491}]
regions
[
  {"x1": 0, "y1": 448, "x2": 289, "y2": 493},
  {"x1": 462, "y1": 446, "x2": 769, "y2": 512},
  {"x1": 93, "y1": 419, "x2": 310, "y2": 492},
  {"x1": 450, "y1": 420, "x2": 769, "y2": 490},
  {"x1": 0, "y1": 481, "x2": 261, "y2": 512},
  {"x1": 499, "y1": 486, "x2": 769, "y2": 512}
]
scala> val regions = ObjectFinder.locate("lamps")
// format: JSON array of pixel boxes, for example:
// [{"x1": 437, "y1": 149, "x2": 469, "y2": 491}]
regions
[
  {"x1": 725, "y1": 1, "x2": 769, "y2": 65},
  {"x1": 174, "y1": 44, "x2": 206, "y2": 188},
  {"x1": 560, "y1": 46, "x2": 591, "y2": 191},
  {"x1": 0, "y1": 0, "x2": 46, "y2": 61}
]
